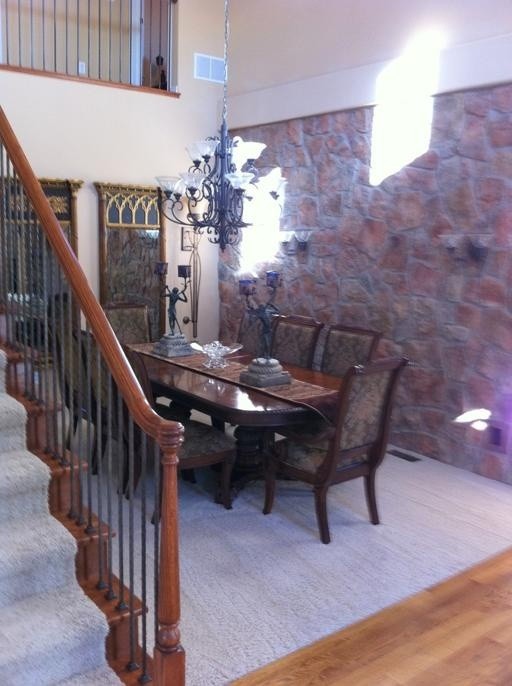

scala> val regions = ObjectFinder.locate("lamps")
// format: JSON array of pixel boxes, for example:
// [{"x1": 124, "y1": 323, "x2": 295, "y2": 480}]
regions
[{"x1": 156, "y1": 1, "x2": 288, "y2": 253}]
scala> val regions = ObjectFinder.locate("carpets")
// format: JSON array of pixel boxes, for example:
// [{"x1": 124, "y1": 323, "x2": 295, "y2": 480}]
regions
[{"x1": 57, "y1": 405, "x2": 510, "y2": 686}]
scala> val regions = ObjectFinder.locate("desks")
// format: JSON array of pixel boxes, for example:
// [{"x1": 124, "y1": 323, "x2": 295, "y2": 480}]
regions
[{"x1": 123, "y1": 342, "x2": 344, "y2": 503}]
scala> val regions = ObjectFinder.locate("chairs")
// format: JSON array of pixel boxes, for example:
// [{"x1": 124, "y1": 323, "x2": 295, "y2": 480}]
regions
[
  {"x1": 269, "y1": 315, "x2": 407, "y2": 377},
  {"x1": 55, "y1": 303, "x2": 237, "y2": 522},
  {"x1": 259, "y1": 356, "x2": 408, "y2": 543}
]
